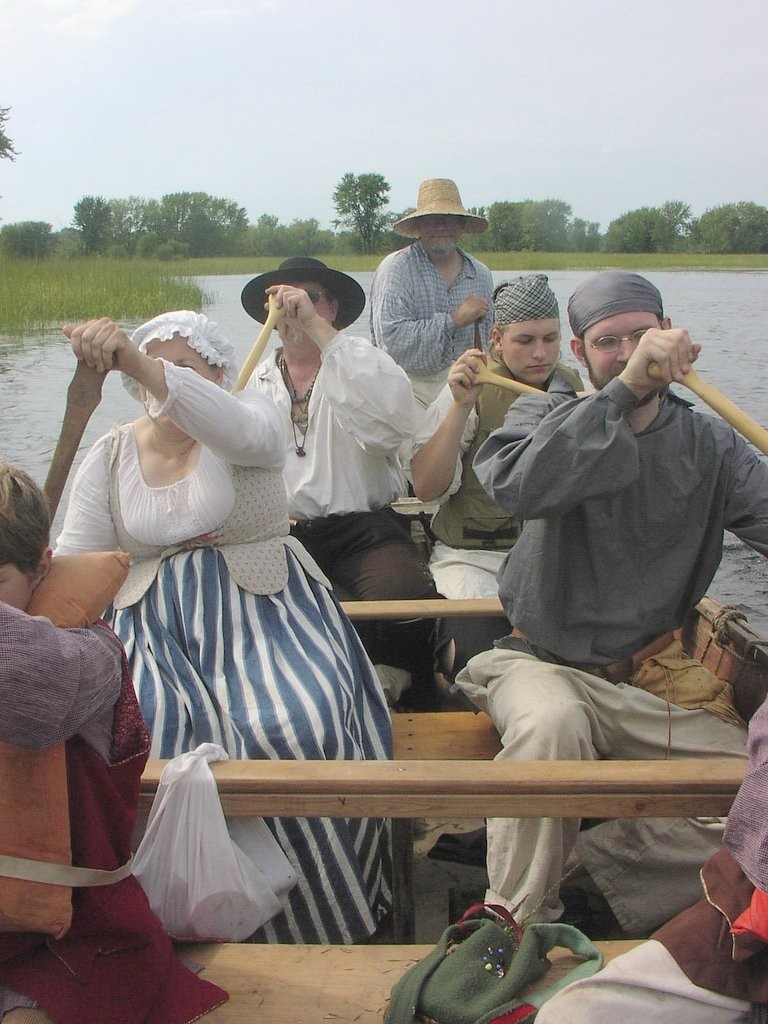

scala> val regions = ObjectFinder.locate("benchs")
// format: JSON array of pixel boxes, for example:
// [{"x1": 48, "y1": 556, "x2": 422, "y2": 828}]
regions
[
  {"x1": 387, "y1": 713, "x2": 504, "y2": 761},
  {"x1": 0, "y1": 941, "x2": 652, "y2": 1024}
]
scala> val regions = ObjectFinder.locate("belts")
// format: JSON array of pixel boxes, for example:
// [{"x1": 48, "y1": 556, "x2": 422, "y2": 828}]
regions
[{"x1": 512, "y1": 626, "x2": 676, "y2": 684}]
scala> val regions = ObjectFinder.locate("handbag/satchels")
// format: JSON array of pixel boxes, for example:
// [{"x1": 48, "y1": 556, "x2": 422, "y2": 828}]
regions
[{"x1": 383, "y1": 919, "x2": 602, "y2": 1024}]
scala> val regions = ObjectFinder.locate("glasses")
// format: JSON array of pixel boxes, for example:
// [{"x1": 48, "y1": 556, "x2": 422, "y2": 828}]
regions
[
  {"x1": 588, "y1": 330, "x2": 647, "y2": 353},
  {"x1": 307, "y1": 288, "x2": 330, "y2": 304}
]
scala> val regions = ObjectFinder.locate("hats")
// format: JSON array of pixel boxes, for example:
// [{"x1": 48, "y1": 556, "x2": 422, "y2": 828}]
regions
[
  {"x1": 393, "y1": 177, "x2": 489, "y2": 240},
  {"x1": 240, "y1": 256, "x2": 366, "y2": 330}
]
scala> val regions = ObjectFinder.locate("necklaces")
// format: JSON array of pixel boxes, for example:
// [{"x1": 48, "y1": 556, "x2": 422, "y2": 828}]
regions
[{"x1": 279, "y1": 353, "x2": 321, "y2": 455}]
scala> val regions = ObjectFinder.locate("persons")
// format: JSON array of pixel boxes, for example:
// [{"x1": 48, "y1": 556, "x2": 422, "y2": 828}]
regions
[
  {"x1": 535, "y1": 697, "x2": 768, "y2": 1024},
  {"x1": 370, "y1": 180, "x2": 496, "y2": 420},
  {"x1": 53, "y1": 309, "x2": 396, "y2": 944},
  {"x1": 382, "y1": 275, "x2": 768, "y2": 1024},
  {"x1": 0, "y1": 464, "x2": 228, "y2": 1024},
  {"x1": 410, "y1": 274, "x2": 584, "y2": 700},
  {"x1": 241, "y1": 258, "x2": 435, "y2": 712}
]
